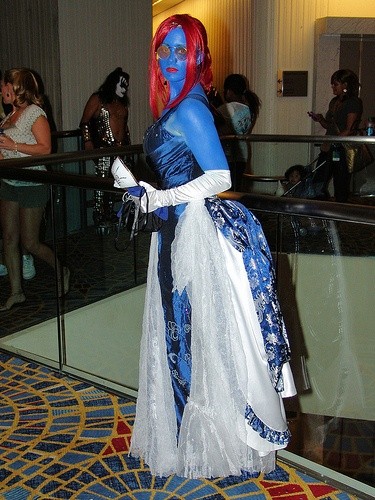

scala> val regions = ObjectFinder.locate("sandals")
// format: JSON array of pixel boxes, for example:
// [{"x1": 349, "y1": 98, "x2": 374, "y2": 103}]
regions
[
  {"x1": 0, "y1": 289, "x2": 26, "y2": 312},
  {"x1": 58, "y1": 266, "x2": 70, "y2": 296}
]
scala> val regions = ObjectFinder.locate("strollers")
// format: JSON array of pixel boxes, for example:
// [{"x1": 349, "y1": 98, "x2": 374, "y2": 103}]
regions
[{"x1": 279, "y1": 144, "x2": 329, "y2": 238}]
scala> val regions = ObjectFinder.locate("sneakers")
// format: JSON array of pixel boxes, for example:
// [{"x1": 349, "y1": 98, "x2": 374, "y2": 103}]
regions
[
  {"x1": 0, "y1": 264, "x2": 8, "y2": 275},
  {"x1": 23, "y1": 254, "x2": 36, "y2": 280}
]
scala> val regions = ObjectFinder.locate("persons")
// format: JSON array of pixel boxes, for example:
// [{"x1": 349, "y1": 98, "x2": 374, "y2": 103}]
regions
[
  {"x1": 124, "y1": 13, "x2": 300, "y2": 478},
  {"x1": 79, "y1": 66, "x2": 136, "y2": 231},
  {"x1": 0, "y1": 67, "x2": 71, "y2": 311},
  {"x1": 216, "y1": 73, "x2": 261, "y2": 191},
  {"x1": 285, "y1": 69, "x2": 364, "y2": 204}
]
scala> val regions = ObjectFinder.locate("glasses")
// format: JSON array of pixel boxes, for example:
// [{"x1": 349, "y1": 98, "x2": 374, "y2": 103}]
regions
[{"x1": 154, "y1": 45, "x2": 201, "y2": 61}]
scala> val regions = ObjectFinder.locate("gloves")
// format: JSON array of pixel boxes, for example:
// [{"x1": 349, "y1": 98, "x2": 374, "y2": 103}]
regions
[{"x1": 130, "y1": 181, "x2": 161, "y2": 213}]
